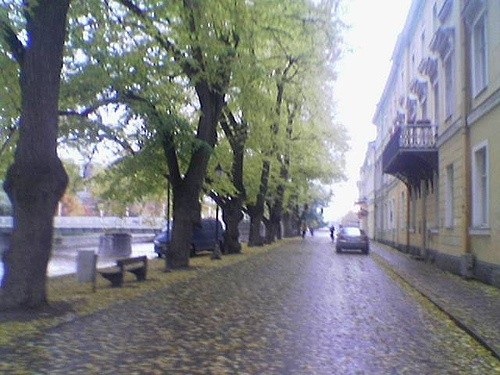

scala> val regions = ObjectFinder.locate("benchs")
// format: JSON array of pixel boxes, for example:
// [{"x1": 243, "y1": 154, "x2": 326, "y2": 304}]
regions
[{"x1": 99, "y1": 256, "x2": 148, "y2": 287}]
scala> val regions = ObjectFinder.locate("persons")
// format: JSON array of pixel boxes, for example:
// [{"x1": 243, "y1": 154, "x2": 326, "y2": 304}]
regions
[
  {"x1": 330, "y1": 225, "x2": 335, "y2": 238},
  {"x1": 302, "y1": 223, "x2": 307, "y2": 237},
  {"x1": 309, "y1": 224, "x2": 313, "y2": 235}
]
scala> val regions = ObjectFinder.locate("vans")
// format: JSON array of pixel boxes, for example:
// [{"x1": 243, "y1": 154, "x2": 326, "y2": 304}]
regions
[{"x1": 153, "y1": 217, "x2": 225, "y2": 259}]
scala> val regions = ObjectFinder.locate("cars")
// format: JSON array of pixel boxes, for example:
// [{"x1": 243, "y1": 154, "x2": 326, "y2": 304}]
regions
[{"x1": 336, "y1": 227, "x2": 369, "y2": 254}]
